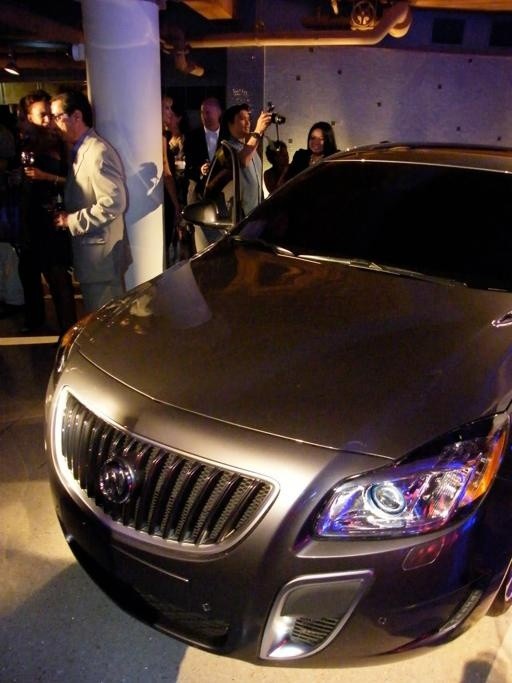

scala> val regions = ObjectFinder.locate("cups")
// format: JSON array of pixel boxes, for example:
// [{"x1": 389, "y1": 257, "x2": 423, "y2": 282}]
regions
[{"x1": 21, "y1": 151, "x2": 34, "y2": 166}]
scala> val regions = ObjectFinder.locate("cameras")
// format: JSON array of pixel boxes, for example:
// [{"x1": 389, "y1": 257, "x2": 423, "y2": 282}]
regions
[{"x1": 267, "y1": 105, "x2": 286, "y2": 125}]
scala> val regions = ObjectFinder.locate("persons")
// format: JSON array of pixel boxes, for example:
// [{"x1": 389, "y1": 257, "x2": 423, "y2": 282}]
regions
[
  {"x1": 158, "y1": 97, "x2": 339, "y2": 260},
  {"x1": 1, "y1": 89, "x2": 133, "y2": 335}
]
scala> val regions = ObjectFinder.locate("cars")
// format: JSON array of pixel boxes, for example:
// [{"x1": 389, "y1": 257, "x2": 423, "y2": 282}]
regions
[{"x1": 40, "y1": 141, "x2": 512, "y2": 672}]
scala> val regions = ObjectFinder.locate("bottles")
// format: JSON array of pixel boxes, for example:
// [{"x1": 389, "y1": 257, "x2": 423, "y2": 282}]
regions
[{"x1": 54, "y1": 193, "x2": 66, "y2": 233}]
